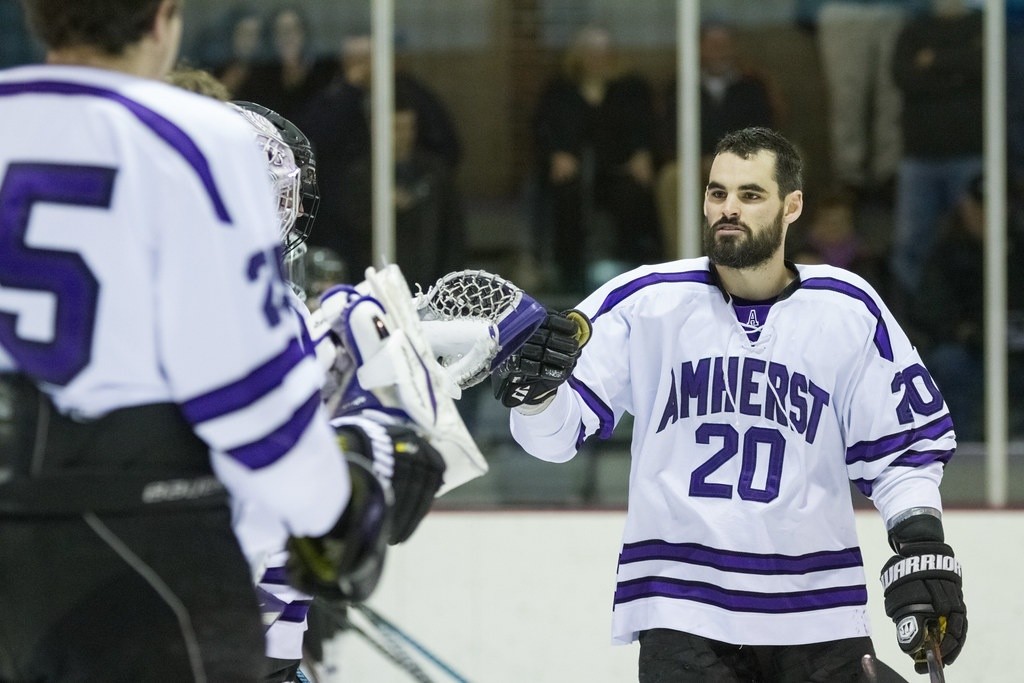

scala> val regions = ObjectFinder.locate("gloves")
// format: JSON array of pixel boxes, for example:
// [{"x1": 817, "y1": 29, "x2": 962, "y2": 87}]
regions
[
  {"x1": 285, "y1": 460, "x2": 391, "y2": 601},
  {"x1": 328, "y1": 408, "x2": 446, "y2": 546},
  {"x1": 491, "y1": 309, "x2": 593, "y2": 407},
  {"x1": 879, "y1": 542, "x2": 967, "y2": 674}
]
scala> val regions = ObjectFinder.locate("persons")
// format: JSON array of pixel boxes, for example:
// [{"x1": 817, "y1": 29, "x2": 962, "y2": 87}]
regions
[
  {"x1": 491, "y1": 125, "x2": 969, "y2": 683},
  {"x1": 162, "y1": 0, "x2": 1024, "y2": 545},
  {"x1": 0, "y1": 0, "x2": 456, "y2": 683}
]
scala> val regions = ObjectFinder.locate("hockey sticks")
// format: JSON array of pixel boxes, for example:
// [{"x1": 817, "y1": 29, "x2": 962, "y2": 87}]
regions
[
  {"x1": 923, "y1": 630, "x2": 947, "y2": 683},
  {"x1": 329, "y1": 602, "x2": 470, "y2": 683}
]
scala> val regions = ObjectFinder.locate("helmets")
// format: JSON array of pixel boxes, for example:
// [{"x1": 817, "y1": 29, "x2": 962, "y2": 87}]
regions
[
  {"x1": 231, "y1": 100, "x2": 320, "y2": 258},
  {"x1": 224, "y1": 102, "x2": 301, "y2": 241}
]
